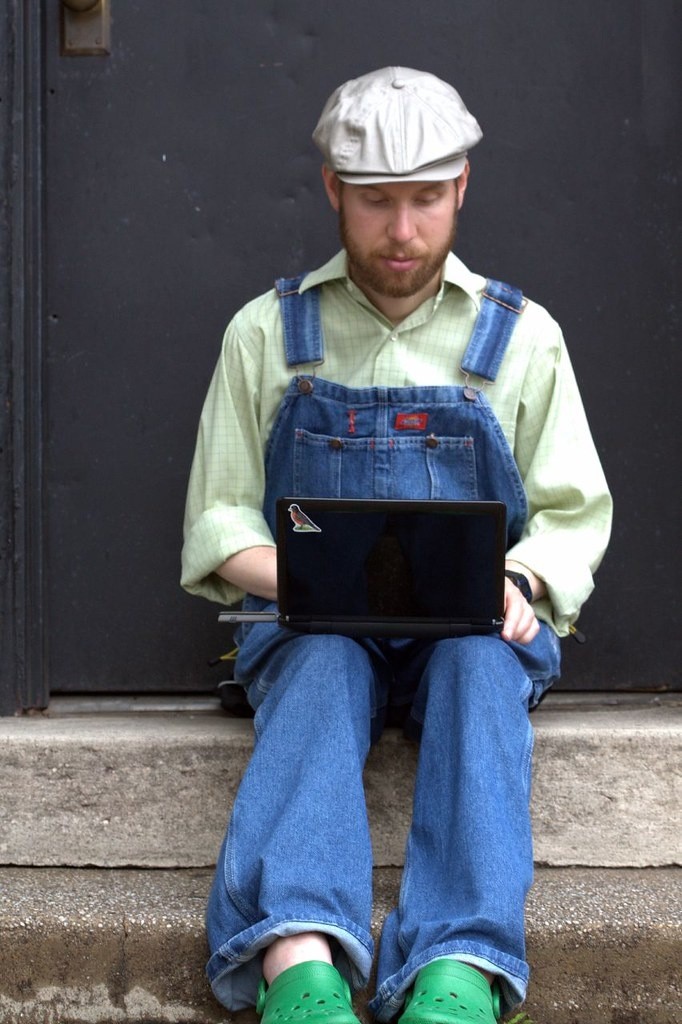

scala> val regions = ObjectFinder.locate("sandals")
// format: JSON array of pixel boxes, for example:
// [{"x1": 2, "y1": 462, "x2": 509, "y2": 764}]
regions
[
  {"x1": 257, "y1": 960, "x2": 359, "y2": 1024},
  {"x1": 398, "y1": 959, "x2": 501, "y2": 1024}
]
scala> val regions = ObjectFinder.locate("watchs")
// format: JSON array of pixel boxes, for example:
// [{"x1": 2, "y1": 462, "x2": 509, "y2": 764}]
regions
[{"x1": 506, "y1": 569, "x2": 533, "y2": 603}]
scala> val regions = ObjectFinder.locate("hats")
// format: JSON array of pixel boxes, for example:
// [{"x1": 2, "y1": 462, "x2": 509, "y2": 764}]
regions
[{"x1": 311, "y1": 65, "x2": 484, "y2": 184}]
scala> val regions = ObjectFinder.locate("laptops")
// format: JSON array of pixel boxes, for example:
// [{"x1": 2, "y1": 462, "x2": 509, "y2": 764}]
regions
[{"x1": 274, "y1": 497, "x2": 505, "y2": 640}]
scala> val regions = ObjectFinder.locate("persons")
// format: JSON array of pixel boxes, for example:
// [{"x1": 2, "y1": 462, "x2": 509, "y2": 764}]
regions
[{"x1": 180, "y1": 68, "x2": 613, "y2": 1024}]
